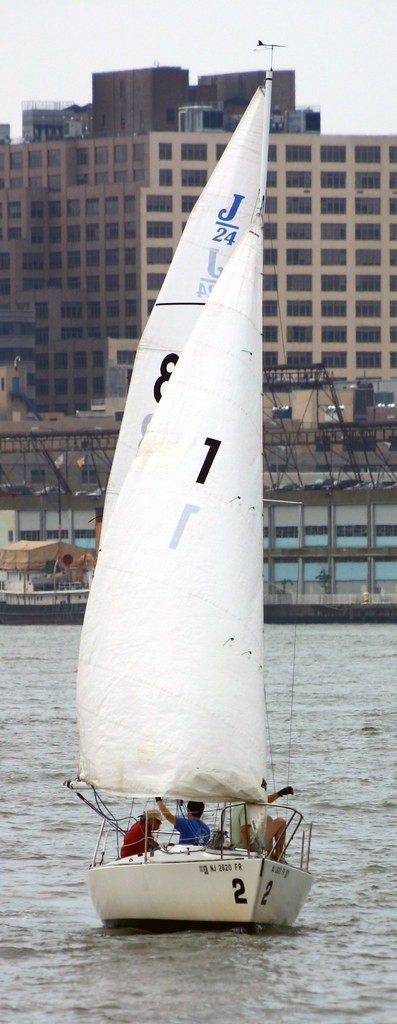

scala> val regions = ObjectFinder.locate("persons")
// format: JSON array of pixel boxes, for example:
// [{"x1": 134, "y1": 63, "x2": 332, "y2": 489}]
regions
[
  {"x1": 155, "y1": 797, "x2": 210, "y2": 846},
  {"x1": 120, "y1": 808, "x2": 163, "y2": 858},
  {"x1": 232, "y1": 778, "x2": 294, "y2": 864}
]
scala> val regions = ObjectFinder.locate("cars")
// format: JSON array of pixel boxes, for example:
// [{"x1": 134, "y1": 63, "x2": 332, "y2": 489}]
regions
[{"x1": 0, "y1": 476, "x2": 397, "y2": 496}]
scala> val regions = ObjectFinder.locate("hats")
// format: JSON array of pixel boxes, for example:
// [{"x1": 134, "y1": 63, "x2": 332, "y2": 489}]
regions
[{"x1": 145, "y1": 808, "x2": 165, "y2": 823}]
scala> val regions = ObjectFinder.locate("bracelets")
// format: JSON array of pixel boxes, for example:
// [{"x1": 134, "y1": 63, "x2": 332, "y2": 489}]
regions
[{"x1": 155, "y1": 797, "x2": 162, "y2": 802}]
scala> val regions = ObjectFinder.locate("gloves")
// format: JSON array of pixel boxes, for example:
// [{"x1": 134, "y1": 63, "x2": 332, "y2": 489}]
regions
[{"x1": 278, "y1": 787, "x2": 293, "y2": 797}]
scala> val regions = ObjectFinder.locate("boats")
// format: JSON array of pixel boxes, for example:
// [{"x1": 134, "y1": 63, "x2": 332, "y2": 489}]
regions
[{"x1": 0, "y1": 540, "x2": 99, "y2": 626}]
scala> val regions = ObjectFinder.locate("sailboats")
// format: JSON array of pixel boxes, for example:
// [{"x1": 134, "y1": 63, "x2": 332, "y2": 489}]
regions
[{"x1": 60, "y1": 37, "x2": 323, "y2": 932}]
